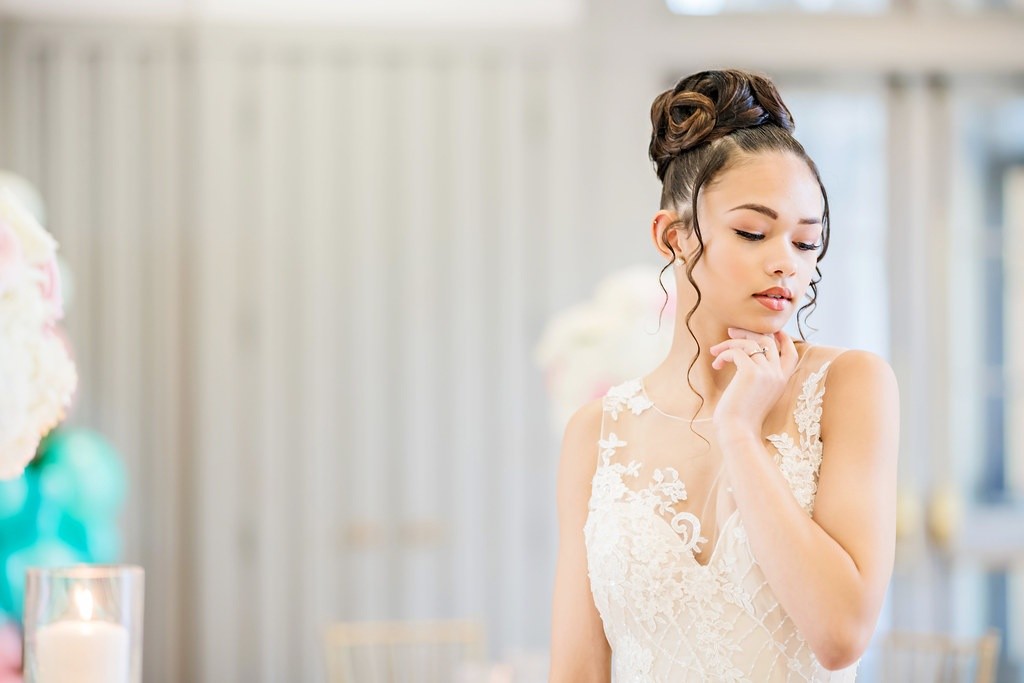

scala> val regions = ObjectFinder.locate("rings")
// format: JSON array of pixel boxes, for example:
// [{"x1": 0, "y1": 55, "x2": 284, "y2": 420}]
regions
[{"x1": 749, "y1": 347, "x2": 768, "y2": 358}]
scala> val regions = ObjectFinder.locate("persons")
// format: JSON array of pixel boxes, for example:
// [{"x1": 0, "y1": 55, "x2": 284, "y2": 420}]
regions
[{"x1": 551, "y1": 68, "x2": 900, "y2": 683}]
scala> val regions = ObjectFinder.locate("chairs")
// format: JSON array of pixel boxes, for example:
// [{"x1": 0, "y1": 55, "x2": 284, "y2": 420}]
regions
[
  {"x1": 855, "y1": 629, "x2": 1003, "y2": 683},
  {"x1": 325, "y1": 620, "x2": 486, "y2": 683}
]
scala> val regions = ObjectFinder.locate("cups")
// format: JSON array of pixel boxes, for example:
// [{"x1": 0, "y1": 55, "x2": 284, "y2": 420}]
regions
[{"x1": 22, "y1": 563, "x2": 143, "y2": 683}]
